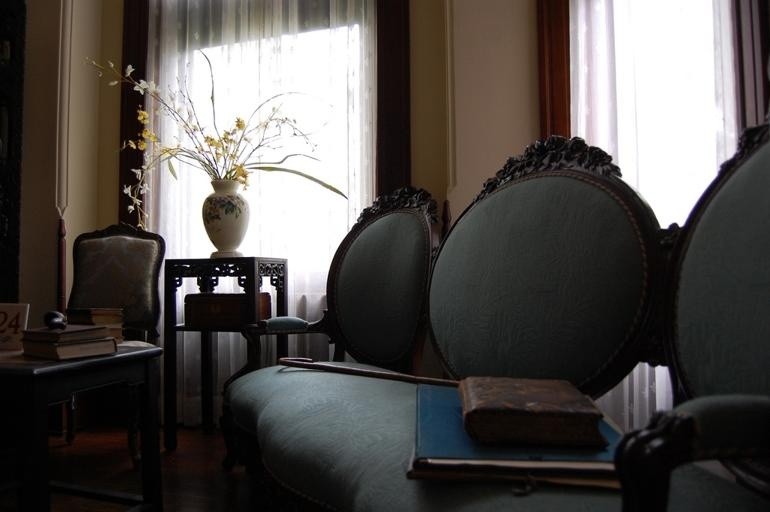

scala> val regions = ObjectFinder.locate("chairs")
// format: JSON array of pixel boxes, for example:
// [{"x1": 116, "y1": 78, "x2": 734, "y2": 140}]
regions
[
  {"x1": 66, "y1": 219, "x2": 166, "y2": 468},
  {"x1": 220, "y1": 115, "x2": 769, "y2": 510}
]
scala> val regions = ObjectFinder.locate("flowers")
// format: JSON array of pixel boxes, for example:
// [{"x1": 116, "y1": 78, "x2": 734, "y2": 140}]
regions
[{"x1": 80, "y1": 47, "x2": 349, "y2": 235}]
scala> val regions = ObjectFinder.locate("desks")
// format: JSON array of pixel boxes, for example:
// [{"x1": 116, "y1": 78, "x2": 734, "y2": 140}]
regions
[
  {"x1": 163, "y1": 257, "x2": 287, "y2": 466},
  {"x1": 1, "y1": 340, "x2": 163, "y2": 511}
]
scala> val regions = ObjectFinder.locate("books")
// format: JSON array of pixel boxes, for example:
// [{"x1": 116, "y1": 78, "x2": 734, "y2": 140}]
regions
[
  {"x1": 21, "y1": 323, "x2": 106, "y2": 342},
  {"x1": 414, "y1": 381, "x2": 629, "y2": 471},
  {"x1": 457, "y1": 375, "x2": 610, "y2": 449},
  {"x1": 65, "y1": 307, "x2": 125, "y2": 344},
  {"x1": 20, "y1": 337, "x2": 118, "y2": 362},
  {"x1": 406, "y1": 446, "x2": 624, "y2": 494}
]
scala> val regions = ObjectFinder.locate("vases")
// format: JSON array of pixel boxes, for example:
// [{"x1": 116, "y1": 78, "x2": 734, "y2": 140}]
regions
[{"x1": 198, "y1": 180, "x2": 252, "y2": 259}]
